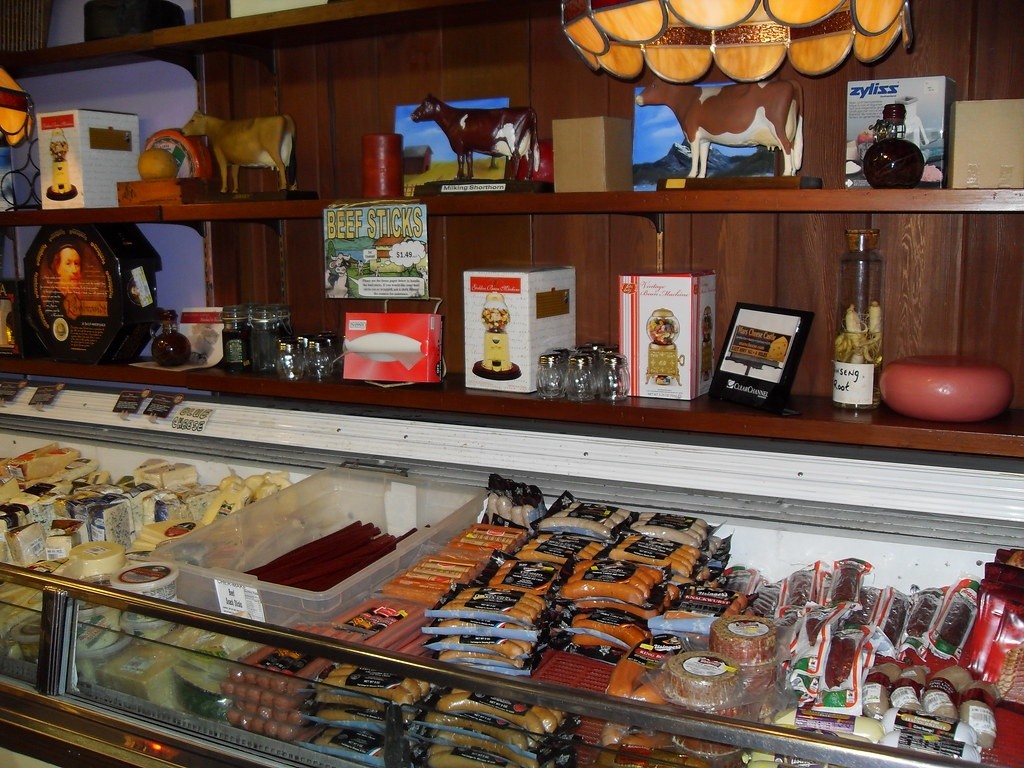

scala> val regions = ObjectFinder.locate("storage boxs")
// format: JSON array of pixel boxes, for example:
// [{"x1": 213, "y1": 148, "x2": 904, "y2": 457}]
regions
[
  {"x1": 552, "y1": 116, "x2": 634, "y2": 192},
  {"x1": 342, "y1": 311, "x2": 442, "y2": 384},
  {"x1": 464, "y1": 265, "x2": 576, "y2": 394},
  {"x1": 844, "y1": 76, "x2": 1024, "y2": 189},
  {"x1": 36, "y1": 109, "x2": 142, "y2": 210},
  {"x1": 148, "y1": 465, "x2": 490, "y2": 631},
  {"x1": 617, "y1": 271, "x2": 718, "y2": 401},
  {"x1": 116, "y1": 176, "x2": 206, "y2": 205}
]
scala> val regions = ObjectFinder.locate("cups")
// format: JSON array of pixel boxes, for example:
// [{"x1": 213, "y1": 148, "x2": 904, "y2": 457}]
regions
[{"x1": 279, "y1": 332, "x2": 340, "y2": 381}]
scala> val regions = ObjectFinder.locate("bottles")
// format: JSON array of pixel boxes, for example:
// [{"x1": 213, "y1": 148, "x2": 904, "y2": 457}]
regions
[{"x1": 536, "y1": 341, "x2": 630, "y2": 402}]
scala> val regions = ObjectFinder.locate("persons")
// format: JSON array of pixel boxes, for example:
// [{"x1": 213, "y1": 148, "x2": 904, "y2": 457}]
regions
[{"x1": 44, "y1": 241, "x2": 81, "y2": 316}]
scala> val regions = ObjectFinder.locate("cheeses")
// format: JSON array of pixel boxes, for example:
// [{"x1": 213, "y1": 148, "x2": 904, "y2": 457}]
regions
[{"x1": 1, "y1": 444, "x2": 298, "y2": 706}]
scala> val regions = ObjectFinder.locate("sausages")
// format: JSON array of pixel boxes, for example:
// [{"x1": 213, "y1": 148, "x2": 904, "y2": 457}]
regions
[{"x1": 219, "y1": 475, "x2": 1024, "y2": 768}]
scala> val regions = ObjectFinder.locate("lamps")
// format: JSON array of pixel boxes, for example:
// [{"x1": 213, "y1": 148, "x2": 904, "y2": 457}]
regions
[
  {"x1": 560, "y1": 0, "x2": 915, "y2": 86},
  {"x1": 0, "y1": 68, "x2": 36, "y2": 148}
]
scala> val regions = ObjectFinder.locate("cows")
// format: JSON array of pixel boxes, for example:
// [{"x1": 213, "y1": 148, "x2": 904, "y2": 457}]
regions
[
  {"x1": 181, "y1": 111, "x2": 297, "y2": 193},
  {"x1": 635, "y1": 74, "x2": 806, "y2": 178},
  {"x1": 409, "y1": 92, "x2": 541, "y2": 178}
]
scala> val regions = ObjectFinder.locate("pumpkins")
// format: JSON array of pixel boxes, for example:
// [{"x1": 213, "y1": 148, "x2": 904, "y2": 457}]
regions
[{"x1": 137, "y1": 147, "x2": 177, "y2": 181}]
